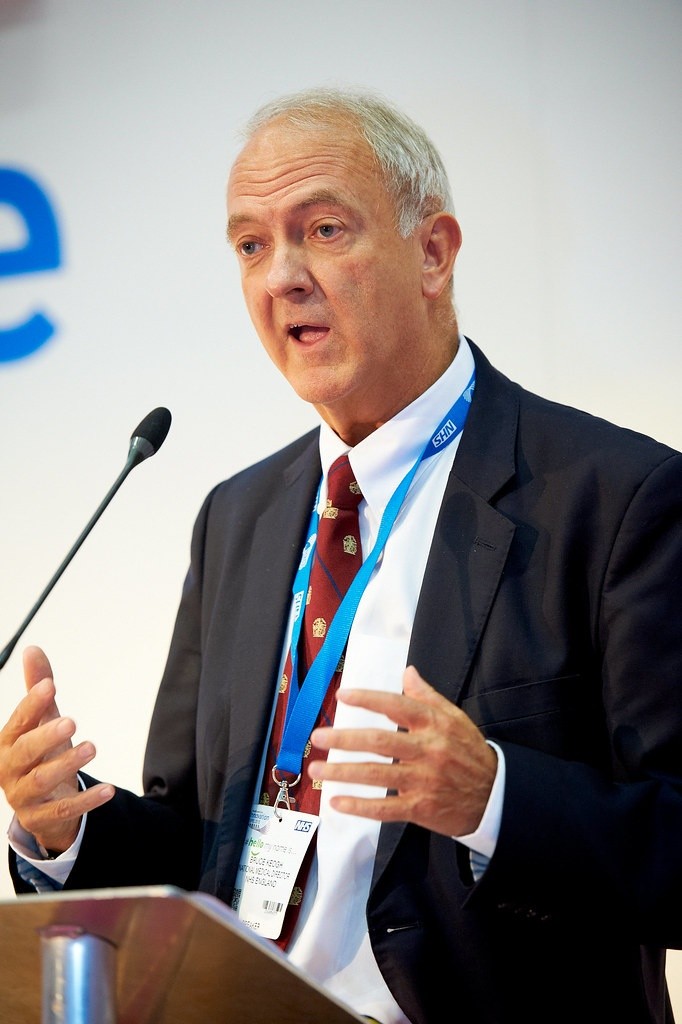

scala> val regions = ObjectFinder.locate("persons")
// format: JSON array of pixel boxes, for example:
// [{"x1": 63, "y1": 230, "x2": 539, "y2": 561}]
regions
[{"x1": 1, "y1": 91, "x2": 682, "y2": 1024}]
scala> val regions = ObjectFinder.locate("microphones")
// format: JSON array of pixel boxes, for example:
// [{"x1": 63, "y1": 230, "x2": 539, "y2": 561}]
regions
[{"x1": 0, "y1": 406, "x2": 172, "y2": 671}]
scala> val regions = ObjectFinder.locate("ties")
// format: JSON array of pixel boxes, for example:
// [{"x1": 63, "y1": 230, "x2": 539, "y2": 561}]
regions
[{"x1": 259, "y1": 455, "x2": 363, "y2": 953}]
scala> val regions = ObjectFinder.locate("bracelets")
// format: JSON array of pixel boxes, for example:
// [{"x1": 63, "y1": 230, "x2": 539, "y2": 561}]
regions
[{"x1": 34, "y1": 836, "x2": 55, "y2": 861}]
